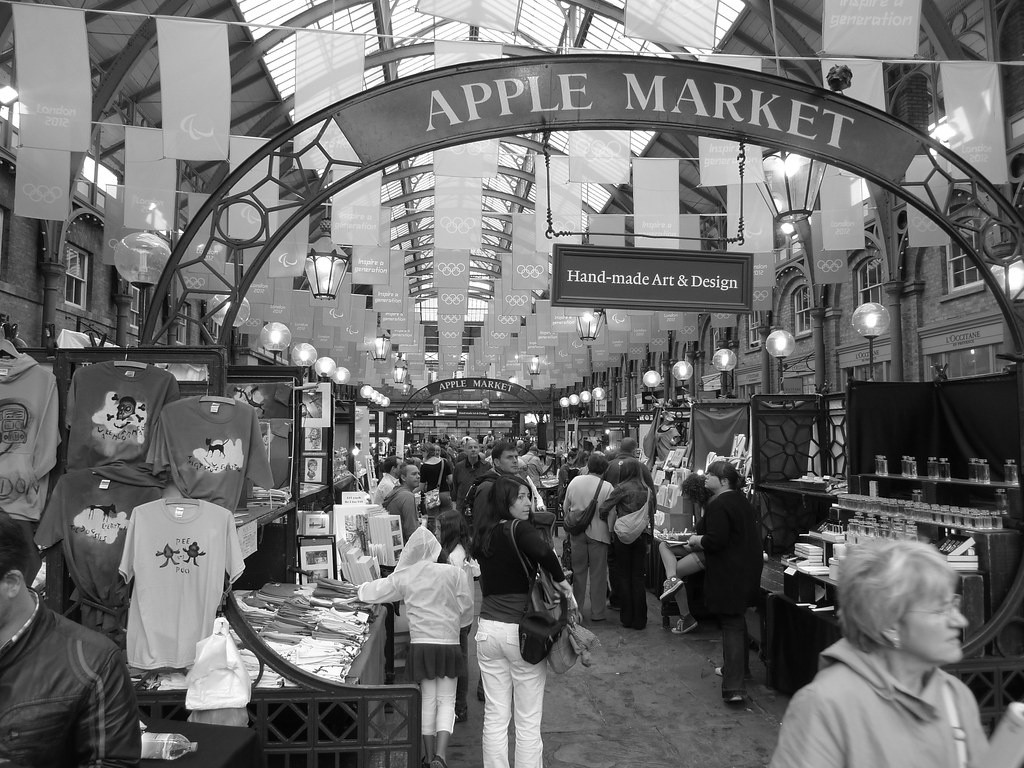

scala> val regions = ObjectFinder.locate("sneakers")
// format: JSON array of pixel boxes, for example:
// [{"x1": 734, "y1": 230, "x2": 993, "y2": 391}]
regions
[
  {"x1": 660, "y1": 576, "x2": 684, "y2": 601},
  {"x1": 670, "y1": 612, "x2": 697, "y2": 634}
]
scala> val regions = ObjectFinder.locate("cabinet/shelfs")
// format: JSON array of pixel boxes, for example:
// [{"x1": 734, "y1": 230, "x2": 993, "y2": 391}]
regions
[{"x1": 755, "y1": 466, "x2": 1024, "y2": 690}]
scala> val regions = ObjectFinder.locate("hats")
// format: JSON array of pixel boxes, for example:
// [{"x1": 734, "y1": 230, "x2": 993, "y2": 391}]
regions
[{"x1": 530, "y1": 446, "x2": 539, "y2": 452}]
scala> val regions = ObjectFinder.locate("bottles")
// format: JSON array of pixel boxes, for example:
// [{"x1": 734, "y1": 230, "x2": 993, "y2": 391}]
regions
[
  {"x1": 141, "y1": 731, "x2": 198, "y2": 760},
  {"x1": 465, "y1": 508, "x2": 472, "y2": 524},
  {"x1": 861, "y1": 514, "x2": 876, "y2": 538},
  {"x1": 847, "y1": 512, "x2": 865, "y2": 536},
  {"x1": 825, "y1": 519, "x2": 843, "y2": 534},
  {"x1": 928, "y1": 457, "x2": 939, "y2": 479},
  {"x1": 950, "y1": 506, "x2": 1002, "y2": 530},
  {"x1": 995, "y1": 489, "x2": 1008, "y2": 515},
  {"x1": 892, "y1": 519, "x2": 904, "y2": 542},
  {"x1": 881, "y1": 497, "x2": 916, "y2": 520},
  {"x1": 1004, "y1": 459, "x2": 1018, "y2": 486},
  {"x1": 906, "y1": 520, "x2": 918, "y2": 543},
  {"x1": 656, "y1": 464, "x2": 662, "y2": 473},
  {"x1": 901, "y1": 456, "x2": 917, "y2": 478},
  {"x1": 965, "y1": 547, "x2": 975, "y2": 556},
  {"x1": 915, "y1": 502, "x2": 950, "y2": 524},
  {"x1": 837, "y1": 494, "x2": 882, "y2": 513},
  {"x1": 875, "y1": 517, "x2": 890, "y2": 540},
  {"x1": 939, "y1": 458, "x2": 950, "y2": 481},
  {"x1": 876, "y1": 455, "x2": 888, "y2": 476},
  {"x1": 765, "y1": 534, "x2": 772, "y2": 556},
  {"x1": 912, "y1": 489, "x2": 923, "y2": 502},
  {"x1": 665, "y1": 463, "x2": 676, "y2": 484},
  {"x1": 968, "y1": 458, "x2": 990, "y2": 483}
]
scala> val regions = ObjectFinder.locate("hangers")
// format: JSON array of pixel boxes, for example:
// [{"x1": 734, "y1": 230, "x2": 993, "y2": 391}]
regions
[{"x1": 0, "y1": 326, "x2": 237, "y2": 506}]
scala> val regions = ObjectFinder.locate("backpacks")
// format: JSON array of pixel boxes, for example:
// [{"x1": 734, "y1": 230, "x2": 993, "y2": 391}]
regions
[{"x1": 462, "y1": 478, "x2": 499, "y2": 540}]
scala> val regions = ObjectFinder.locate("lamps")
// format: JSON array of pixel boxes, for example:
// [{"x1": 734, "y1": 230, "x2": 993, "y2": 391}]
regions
[
  {"x1": 400, "y1": 377, "x2": 412, "y2": 395},
  {"x1": 576, "y1": 182, "x2": 607, "y2": 342},
  {"x1": 559, "y1": 387, "x2": 605, "y2": 415},
  {"x1": 766, "y1": 330, "x2": 795, "y2": 393},
  {"x1": 988, "y1": 254, "x2": 1024, "y2": 304},
  {"x1": 359, "y1": 385, "x2": 390, "y2": 409},
  {"x1": 852, "y1": 302, "x2": 891, "y2": 381},
  {"x1": 643, "y1": 349, "x2": 737, "y2": 403},
  {"x1": 305, "y1": 170, "x2": 353, "y2": 300},
  {"x1": 370, "y1": 313, "x2": 391, "y2": 362},
  {"x1": 391, "y1": 353, "x2": 408, "y2": 384},
  {"x1": 527, "y1": 355, "x2": 541, "y2": 375},
  {"x1": 754, "y1": 0, "x2": 827, "y2": 223},
  {"x1": 261, "y1": 322, "x2": 351, "y2": 393},
  {"x1": 114, "y1": 234, "x2": 251, "y2": 344}
]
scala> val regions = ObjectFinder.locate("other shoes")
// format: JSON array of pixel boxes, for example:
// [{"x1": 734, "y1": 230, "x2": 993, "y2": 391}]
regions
[
  {"x1": 429, "y1": 756, "x2": 447, "y2": 768},
  {"x1": 714, "y1": 667, "x2": 723, "y2": 677},
  {"x1": 477, "y1": 681, "x2": 485, "y2": 701},
  {"x1": 724, "y1": 694, "x2": 747, "y2": 702},
  {"x1": 422, "y1": 756, "x2": 430, "y2": 768},
  {"x1": 455, "y1": 705, "x2": 466, "y2": 723},
  {"x1": 607, "y1": 602, "x2": 621, "y2": 611}
]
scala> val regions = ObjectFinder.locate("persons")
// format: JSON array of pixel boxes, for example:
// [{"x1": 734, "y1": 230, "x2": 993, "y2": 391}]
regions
[
  {"x1": 358, "y1": 527, "x2": 470, "y2": 768},
  {"x1": 659, "y1": 474, "x2": 715, "y2": 634},
  {"x1": 770, "y1": 538, "x2": 988, "y2": 768},
  {"x1": 375, "y1": 431, "x2": 657, "y2": 722},
  {"x1": 688, "y1": 461, "x2": 759, "y2": 707},
  {"x1": 471, "y1": 475, "x2": 579, "y2": 768},
  {"x1": 0, "y1": 513, "x2": 143, "y2": 768}
]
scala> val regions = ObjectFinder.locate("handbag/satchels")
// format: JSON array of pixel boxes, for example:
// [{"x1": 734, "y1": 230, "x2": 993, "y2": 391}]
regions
[
  {"x1": 614, "y1": 500, "x2": 651, "y2": 544},
  {"x1": 563, "y1": 500, "x2": 597, "y2": 536},
  {"x1": 559, "y1": 540, "x2": 573, "y2": 586},
  {"x1": 424, "y1": 487, "x2": 441, "y2": 511},
  {"x1": 519, "y1": 612, "x2": 562, "y2": 664},
  {"x1": 531, "y1": 549, "x2": 583, "y2": 674}
]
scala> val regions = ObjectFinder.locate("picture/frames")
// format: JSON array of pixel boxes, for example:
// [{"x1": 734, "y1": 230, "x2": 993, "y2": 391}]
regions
[
  {"x1": 300, "y1": 383, "x2": 332, "y2": 427},
  {"x1": 652, "y1": 447, "x2": 690, "y2": 527},
  {"x1": 299, "y1": 427, "x2": 403, "y2": 587}
]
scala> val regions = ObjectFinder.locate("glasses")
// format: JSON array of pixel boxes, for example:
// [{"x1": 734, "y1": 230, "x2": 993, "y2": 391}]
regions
[
  {"x1": 518, "y1": 465, "x2": 528, "y2": 471},
  {"x1": 905, "y1": 594, "x2": 963, "y2": 617}
]
